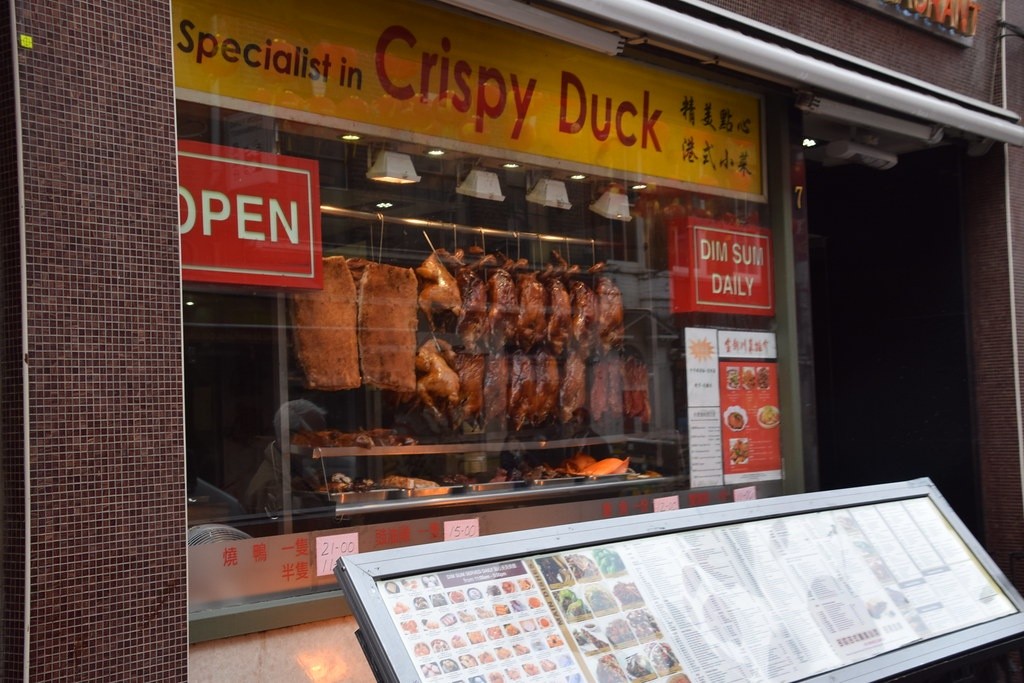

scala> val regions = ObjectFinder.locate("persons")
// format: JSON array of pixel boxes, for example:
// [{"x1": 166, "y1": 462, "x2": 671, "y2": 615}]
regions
[
  {"x1": 567, "y1": 408, "x2": 610, "y2": 482},
  {"x1": 500, "y1": 394, "x2": 570, "y2": 487},
  {"x1": 243, "y1": 400, "x2": 334, "y2": 514}
]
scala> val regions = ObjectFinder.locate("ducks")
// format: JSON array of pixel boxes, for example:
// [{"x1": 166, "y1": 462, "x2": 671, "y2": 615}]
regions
[{"x1": 417, "y1": 251, "x2": 654, "y2": 432}]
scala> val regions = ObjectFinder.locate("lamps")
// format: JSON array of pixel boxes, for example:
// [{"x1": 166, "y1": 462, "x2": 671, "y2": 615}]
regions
[
  {"x1": 438, "y1": 0, "x2": 624, "y2": 58},
  {"x1": 367, "y1": 150, "x2": 420, "y2": 185},
  {"x1": 590, "y1": 191, "x2": 631, "y2": 221},
  {"x1": 525, "y1": 179, "x2": 573, "y2": 210},
  {"x1": 795, "y1": 91, "x2": 942, "y2": 147},
  {"x1": 826, "y1": 141, "x2": 898, "y2": 171},
  {"x1": 455, "y1": 167, "x2": 505, "y2": 202}
]
